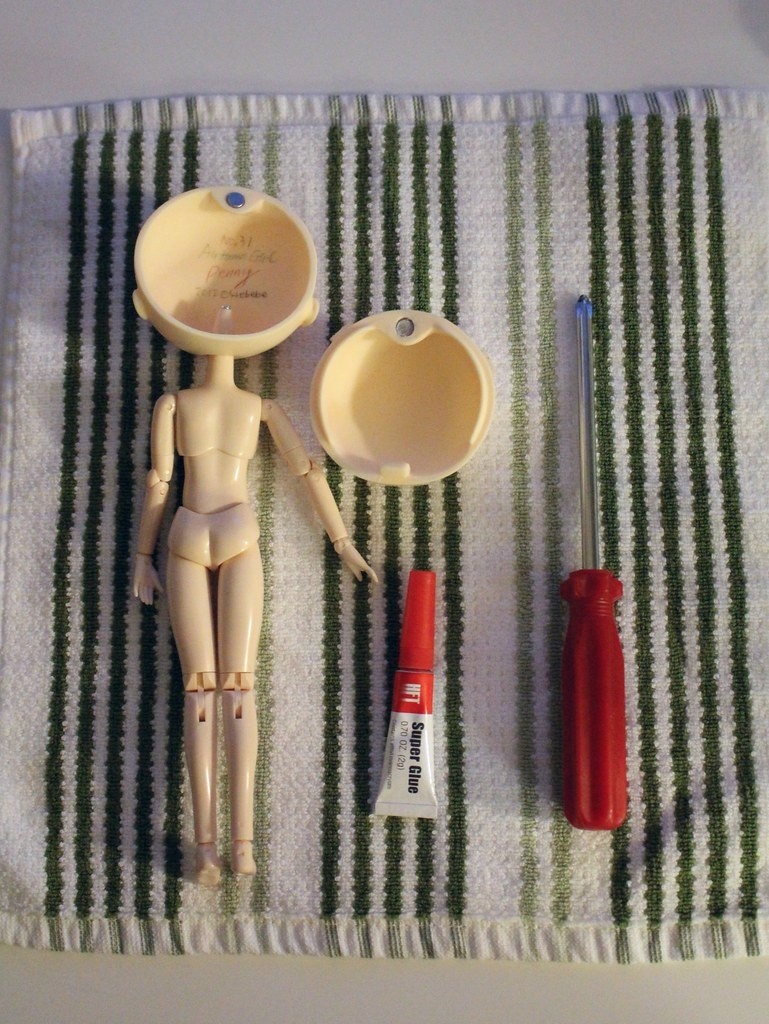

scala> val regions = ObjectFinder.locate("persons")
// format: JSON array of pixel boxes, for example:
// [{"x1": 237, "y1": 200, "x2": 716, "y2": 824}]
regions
[{"x1": 130, "y1": 185, "x2": 382, "y2": 882}]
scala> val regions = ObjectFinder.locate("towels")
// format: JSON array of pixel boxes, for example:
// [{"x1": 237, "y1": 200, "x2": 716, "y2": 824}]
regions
[{"x1": 4, "y1": 89, "x2": 767, "y2": 965}]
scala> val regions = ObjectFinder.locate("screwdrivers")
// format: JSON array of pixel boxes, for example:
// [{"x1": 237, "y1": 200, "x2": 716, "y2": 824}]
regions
[{"x1": 562, "y1": 292, "x2": 630, "y2": 831}]
